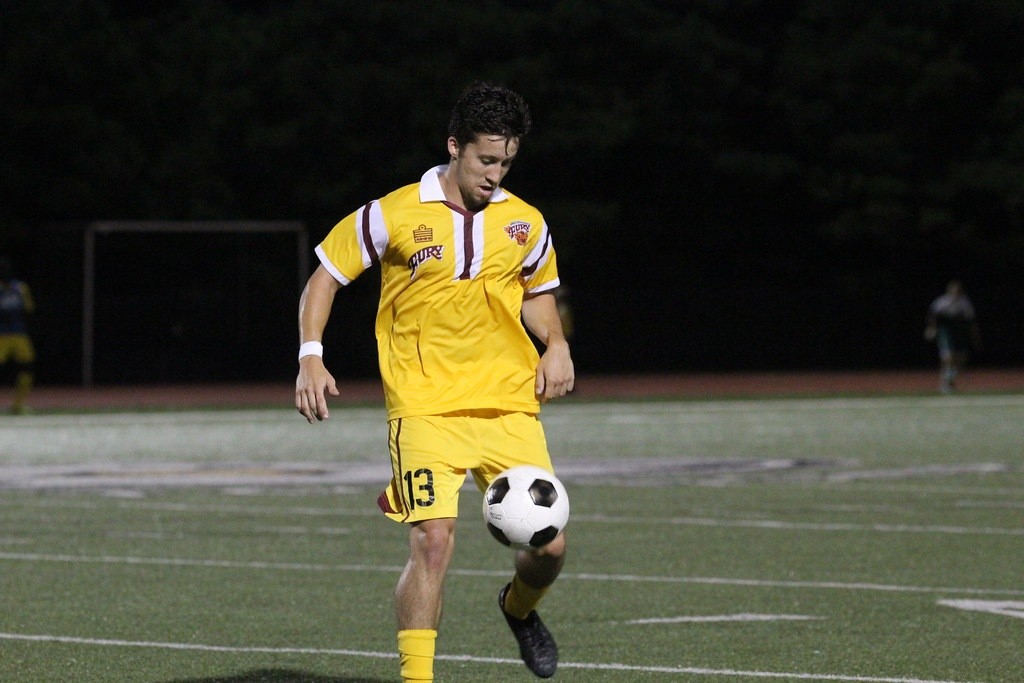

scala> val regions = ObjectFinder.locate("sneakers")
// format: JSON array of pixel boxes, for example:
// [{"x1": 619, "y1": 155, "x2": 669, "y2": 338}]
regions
[{"x1": 499, "y1": 582, "x2": 559, "y2": 678}]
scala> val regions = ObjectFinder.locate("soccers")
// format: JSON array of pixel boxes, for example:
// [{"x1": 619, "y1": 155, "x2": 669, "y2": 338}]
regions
[{"x1": 482, "y1": 468, "x2": 568, "y2": 549}]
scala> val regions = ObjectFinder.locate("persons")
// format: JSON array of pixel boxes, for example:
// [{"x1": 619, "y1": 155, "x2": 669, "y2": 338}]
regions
[
  {"x1": 295, "y1": 81, "x2": 576, "y2": 683},
  {"x1": 0, "y1": 261, "x2": 36, "y2": 415},
  {"x1": 925, "y1": 278, "x2": 982, "y2": 394}
]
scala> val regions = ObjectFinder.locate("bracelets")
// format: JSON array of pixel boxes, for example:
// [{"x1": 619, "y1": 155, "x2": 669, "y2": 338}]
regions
[{"x1": 298, "y1": 341, "x2": 323, "y2": 360}]
